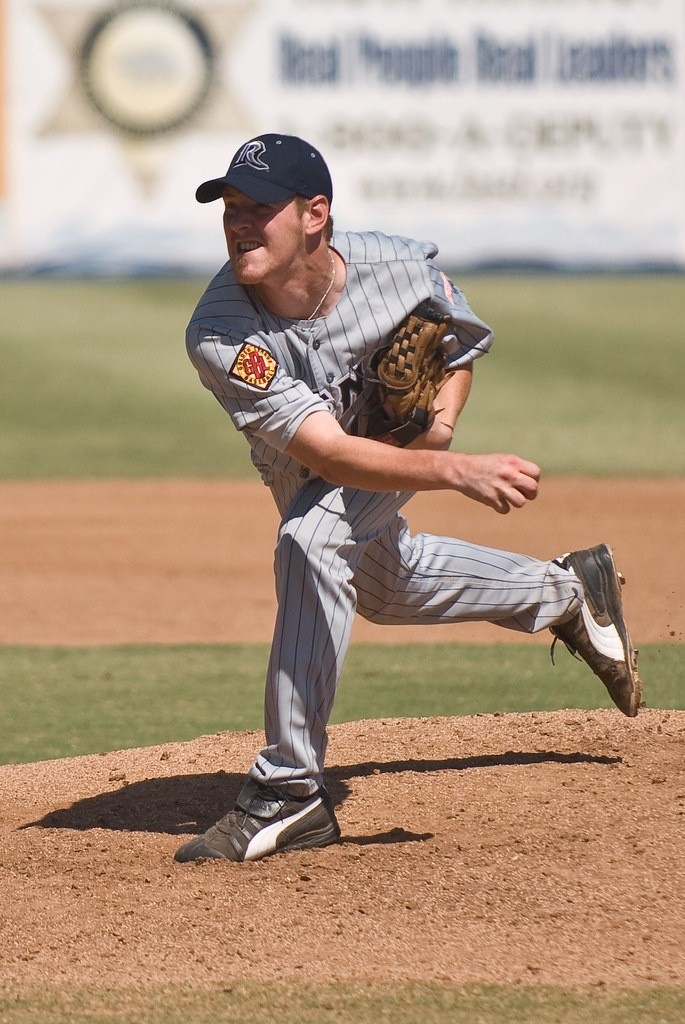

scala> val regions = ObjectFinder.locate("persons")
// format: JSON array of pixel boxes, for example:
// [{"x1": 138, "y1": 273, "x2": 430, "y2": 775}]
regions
[{"x1": 173, "y1": 133, "x2": 641, "y2": 864}]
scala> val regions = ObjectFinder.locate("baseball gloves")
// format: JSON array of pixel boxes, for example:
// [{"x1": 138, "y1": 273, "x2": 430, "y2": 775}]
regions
[{"x1": 376, "y1": 302, "x2": 452, "y2": 439}]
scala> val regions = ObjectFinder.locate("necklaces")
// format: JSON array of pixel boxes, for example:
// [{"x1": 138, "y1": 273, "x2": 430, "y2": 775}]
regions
[{"x1": 307, "y1": 248, "x2": 335, "y2": 320}]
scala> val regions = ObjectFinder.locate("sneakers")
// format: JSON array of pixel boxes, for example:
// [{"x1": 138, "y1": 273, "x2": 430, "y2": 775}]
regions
[
  {"x1": 546, "y1": 543, "x2": 641, "y2": 717},
  {"x1": 174, "y1": 777, "x2": 342, "y2": 863}
]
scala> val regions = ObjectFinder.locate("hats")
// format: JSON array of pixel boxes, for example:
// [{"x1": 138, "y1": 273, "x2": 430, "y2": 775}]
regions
[{"x1": 195, "y1": 134, "x2": 333, "y2": 208}]
